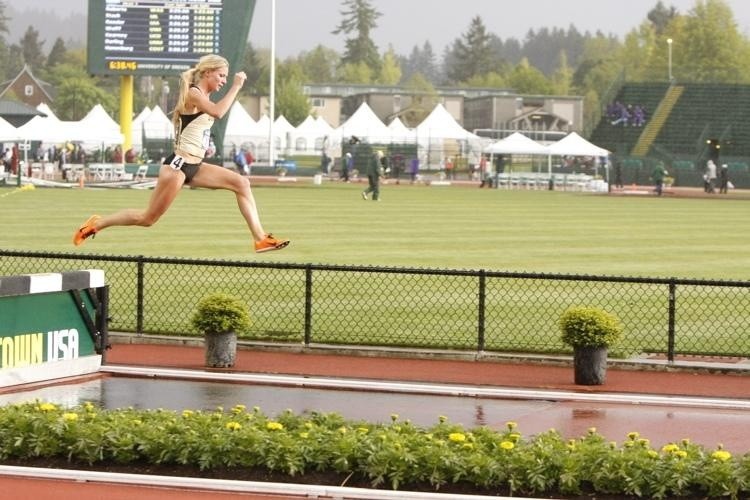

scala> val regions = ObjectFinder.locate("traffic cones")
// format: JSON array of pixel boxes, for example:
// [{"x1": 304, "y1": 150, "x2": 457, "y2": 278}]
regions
[{"x1": 78, "y1": 176, "x2": 85, "y2": 190}]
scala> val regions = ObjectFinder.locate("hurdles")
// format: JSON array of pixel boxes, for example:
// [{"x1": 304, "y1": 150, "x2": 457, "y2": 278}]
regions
[{"x1": 0, "y1": 269, "x2": 109, "y2": 388}]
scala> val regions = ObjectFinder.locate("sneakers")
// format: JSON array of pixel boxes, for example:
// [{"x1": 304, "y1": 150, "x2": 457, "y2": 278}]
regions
[
  {"x1": 256, "y1": 233, "x2": 290, "y2": 253},
  {"x1": 73, "y1": 216, "x2": 99, "y2": 245}
]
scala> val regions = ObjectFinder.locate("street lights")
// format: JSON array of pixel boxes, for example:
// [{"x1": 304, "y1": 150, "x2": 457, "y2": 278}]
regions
[{"x1": 664, "y1": 37, "x2": 676, "y2": 80}]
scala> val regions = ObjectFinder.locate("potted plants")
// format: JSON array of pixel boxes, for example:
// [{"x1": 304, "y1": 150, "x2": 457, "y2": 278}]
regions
[
  {"x1": 190, "y1": 294, "x2": 253, "y2": 368},
  {"x1": 557, "y1": 306, "x2": 626, "y2": 386}
]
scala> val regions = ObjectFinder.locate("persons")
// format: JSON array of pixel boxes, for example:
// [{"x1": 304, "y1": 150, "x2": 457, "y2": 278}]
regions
[
  {"x1": 0, "y1": 136, "x2": 733, "y2": 198},
  {"x1": 71, "y1": 54, "x2": 291, "y2": 255},
  {"x1": 606, "y1": 99, "x2": 648, "y2": 128}
]
